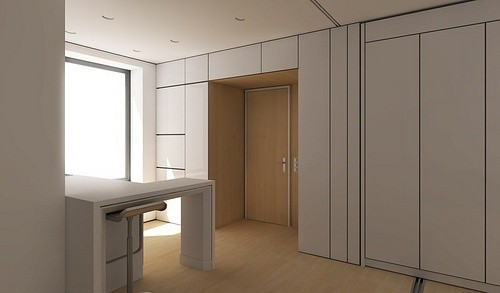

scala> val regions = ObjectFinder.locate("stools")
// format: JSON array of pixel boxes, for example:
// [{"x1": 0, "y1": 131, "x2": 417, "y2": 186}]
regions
[{"x1": 106, "y1": 201, "x2": 167, "y2": 291}]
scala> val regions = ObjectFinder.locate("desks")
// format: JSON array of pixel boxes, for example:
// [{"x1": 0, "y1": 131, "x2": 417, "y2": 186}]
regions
[{"x1": 65, "y1": 177, "x2": 216, "y2": 293}]
